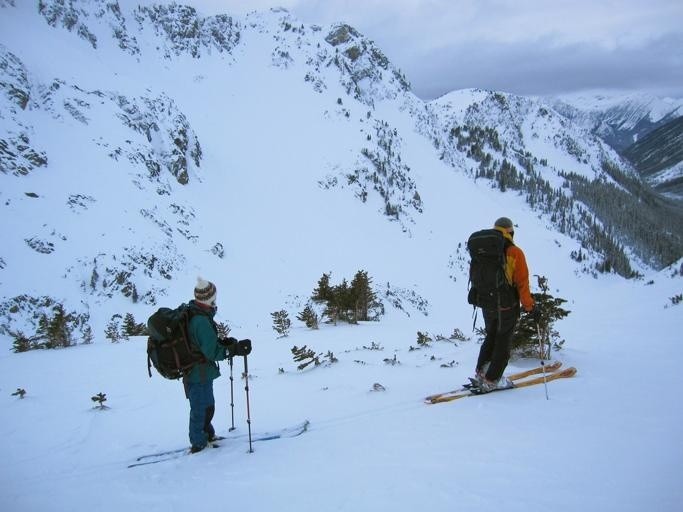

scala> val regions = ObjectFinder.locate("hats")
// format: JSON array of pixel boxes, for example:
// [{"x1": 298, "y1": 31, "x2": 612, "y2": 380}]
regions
[
  {"x1": 193, "y1": 276, "x2": 217, "y2": 305},
  {"x1": 493, "y1": 216, "x2": 515, "y2": 232}
]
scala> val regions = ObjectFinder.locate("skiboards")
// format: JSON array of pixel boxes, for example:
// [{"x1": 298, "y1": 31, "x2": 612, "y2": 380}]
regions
[
  {"x1": 128, "y1": 422, "x2": 309, "y2": 467},
  {"x1": 426, "y1": 361, "x2": 576, "y2": 405}
]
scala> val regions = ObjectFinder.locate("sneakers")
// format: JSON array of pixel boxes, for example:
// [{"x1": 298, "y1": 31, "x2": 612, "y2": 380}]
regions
[
  {"x1": 190, "y1": 436, "x2": 225, "y2": 454},
  {"x1": 476, "y1": 371, "x2": 513, "y2": 393}
]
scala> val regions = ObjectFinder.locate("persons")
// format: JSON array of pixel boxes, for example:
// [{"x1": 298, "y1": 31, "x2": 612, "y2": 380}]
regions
[
  {"x1": 181, "y1": 276, "x2": 253, "y2": 454},
  {"x1": 471, "y1": 217, "x2": 543, "y2": 391}
]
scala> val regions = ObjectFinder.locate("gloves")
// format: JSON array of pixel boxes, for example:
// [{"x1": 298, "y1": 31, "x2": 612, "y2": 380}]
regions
[{"x1": 220, "y1": 337, "x2": 252, "y2": 356}]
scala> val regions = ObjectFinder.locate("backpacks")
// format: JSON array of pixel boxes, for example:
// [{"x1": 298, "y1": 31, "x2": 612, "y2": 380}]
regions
[
  {"x1": 146, "y1": 302, "x2": 209, "y2": 380},
  {"x1": 467, "y1": 229, "x2": 520, "y2": 310}
]
